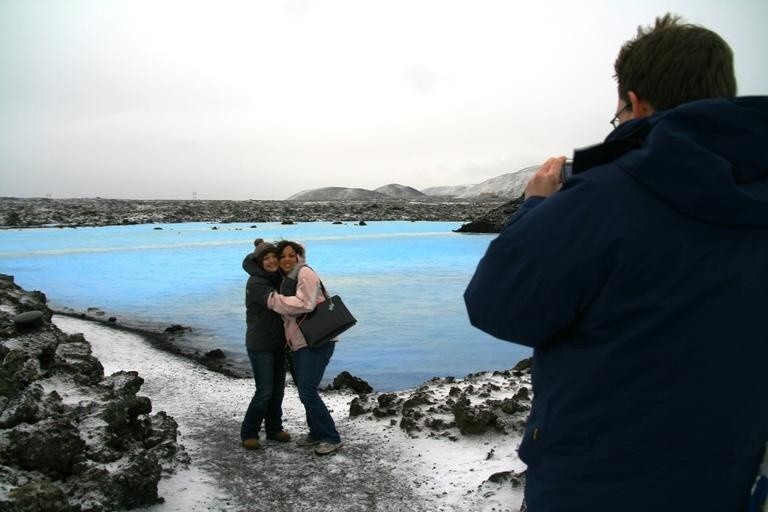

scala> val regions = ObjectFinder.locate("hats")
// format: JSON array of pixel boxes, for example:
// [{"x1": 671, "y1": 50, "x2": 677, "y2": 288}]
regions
[{"x1": 253, "y1": 239, "x2": 277, "y2": 266}]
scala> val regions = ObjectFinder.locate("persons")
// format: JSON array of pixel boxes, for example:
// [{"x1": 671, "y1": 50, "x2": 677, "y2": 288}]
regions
[
  {"x1": 267, "y1": 238, "x2": 344, "y2": 453},
  {"x1": 463, "y1": 13, "x2": 765, "y2": 510},
  {"x1": 239, "y1": 237, "x2": 293, "y2": 450}
]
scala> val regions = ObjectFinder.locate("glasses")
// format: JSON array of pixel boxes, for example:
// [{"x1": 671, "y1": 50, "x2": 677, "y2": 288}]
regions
[{"x1": 610, "y1": 104, "x2": 630, "y2": 129}]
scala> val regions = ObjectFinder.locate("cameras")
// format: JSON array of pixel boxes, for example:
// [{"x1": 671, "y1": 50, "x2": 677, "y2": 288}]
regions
[{"x1": 560, "y1": 158, "x2": 574, "y2": 184}]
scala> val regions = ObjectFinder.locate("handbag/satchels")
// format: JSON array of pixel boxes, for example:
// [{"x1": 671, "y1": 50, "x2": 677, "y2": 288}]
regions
[{"x1": 296, "y1": 296, "x2": 357, "y2": 350}]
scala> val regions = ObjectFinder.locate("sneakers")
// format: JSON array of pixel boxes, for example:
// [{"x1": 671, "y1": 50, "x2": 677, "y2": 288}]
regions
[
  {"x1": 296, "y1": 437, "x2": 320, "y2": 446},
  {"x1": 266, "y1": 432, "x2": 290, "y2": 441},
  {"x1": 243, "y1": 439, "x2": 258, "y2": 450},
  {"x1": 315, "y1": 441, "x2": 344, "y2": 454}
]
